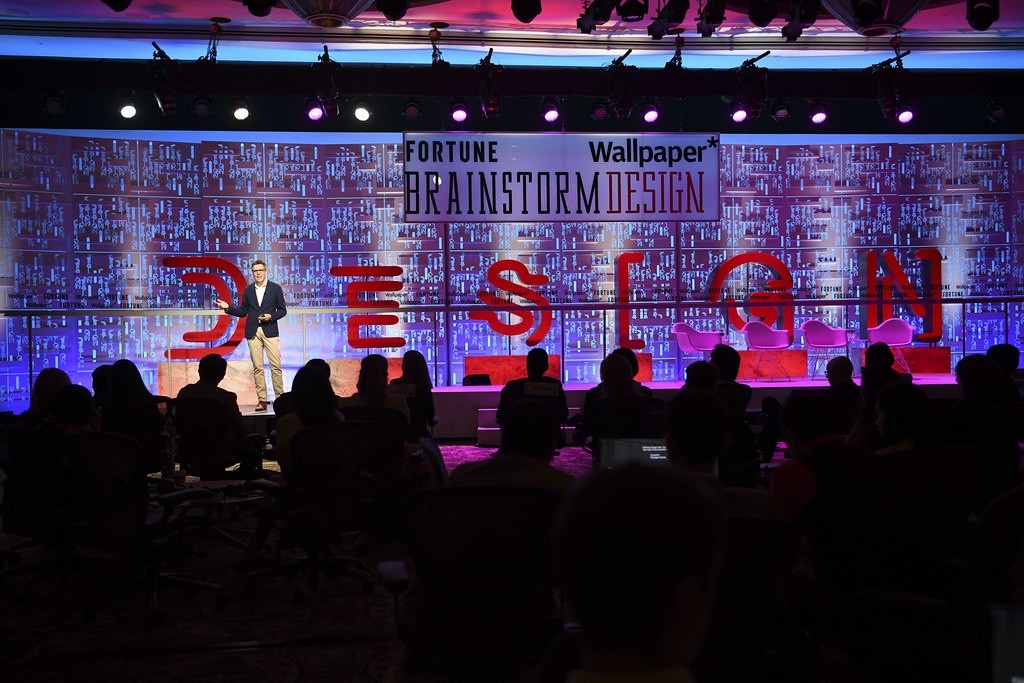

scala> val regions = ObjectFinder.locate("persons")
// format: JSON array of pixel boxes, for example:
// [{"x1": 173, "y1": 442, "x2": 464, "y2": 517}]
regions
[
  {"x1": 943, "y1": 355, "x2": 1024, "y2": 525},
  {"x1": 213, "y1": 260, "x2": 287, "y2": 411},
  {"x1": 445, "y1": 399, "x2": 589, "y2": 654},
  {"x1": 274, "y1": 336, "x2": 916, "y2": 488},
  {"x1": 46, "y1": 385, "x2": 103, "y2": 438},
  {"x1": 173, "y1": 353, "x2": 264, "y2": 483},
  {"x1": 102, "y1": 360, "x2": 181, "y2": 499},
  {"x1": 765, "y1": 386, "x2": 946, "y2": 670},
  {"x1": 91, "y1": 364, "x2": 116, "y2": 404},
  {"x1": 549, "y1": 458, "x2": 728, "y2": 681},
  {"x1": 984, "y1": 345, "x2": 1022, "y2": 400},
  {"x1": 660, "y1": 387, "x2": 773, "y2": 525},
  {"x1": 8, "y1": 367, "x2": 73, "y2": 435},
  {"x1": 869, "y1": 379, "x2": 956, "y2": 474}
]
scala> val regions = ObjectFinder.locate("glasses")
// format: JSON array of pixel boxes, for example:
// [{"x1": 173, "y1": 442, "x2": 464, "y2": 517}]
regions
[{"x1": 252, "y1": 269, "x2": 265, "y2": 272}]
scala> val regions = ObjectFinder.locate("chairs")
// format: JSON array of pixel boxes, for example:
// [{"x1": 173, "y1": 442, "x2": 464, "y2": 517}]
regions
[
  {"x1": 865, "y1": 318, "x2": 914, "y2": 378},
  {"x1": 739, "y1": 321, "x2": 792, "y2": 384},
  {"x1": 799, "y1": 320, "x2": 848, "y2": 381},
  {"x1": 671, "y1": 321, "x2": 724, "y2": 385}
]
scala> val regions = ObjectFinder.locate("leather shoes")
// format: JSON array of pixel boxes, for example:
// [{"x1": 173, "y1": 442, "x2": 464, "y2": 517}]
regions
[{"x1": 255, "y1": 401, "x2": 267, "y2": 411}]
